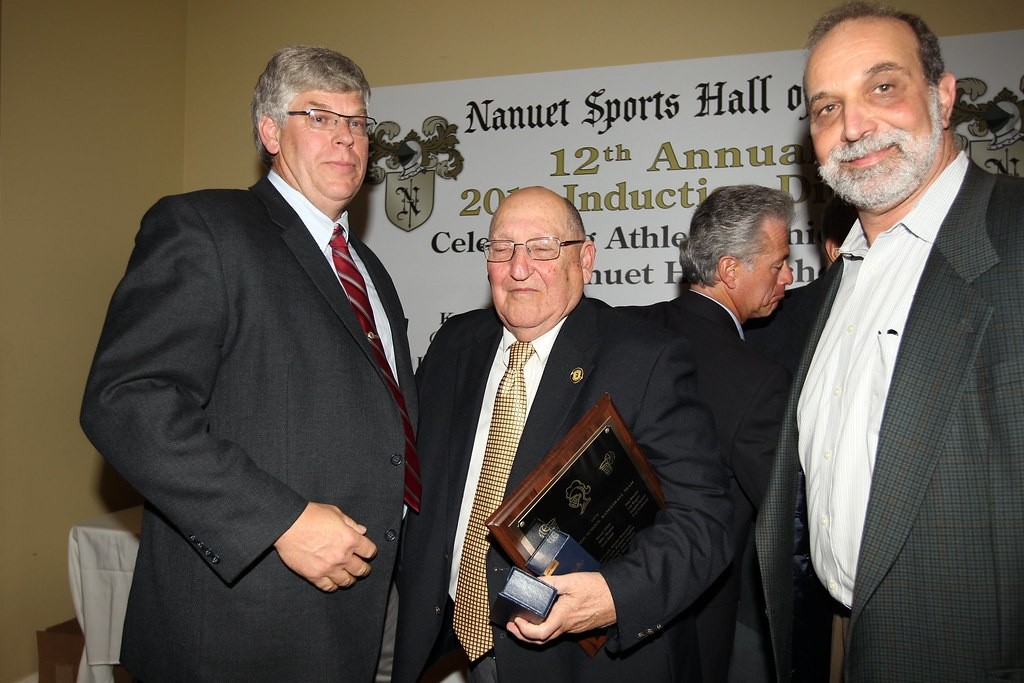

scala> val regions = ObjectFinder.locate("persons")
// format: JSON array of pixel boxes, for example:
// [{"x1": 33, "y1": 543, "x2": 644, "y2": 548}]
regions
[
  {"x1": 78, "y1": 46, "x2": 419, "y2": 683},
  {"x1": 392, "y1": 185, "x2": 752, "y2": 683},
  {"x1": 751, "y1": 0, "x2": 1024, "y2": 683},
  {"x1": 611, "y1": 185, "x2": 860, "y2": 683}
]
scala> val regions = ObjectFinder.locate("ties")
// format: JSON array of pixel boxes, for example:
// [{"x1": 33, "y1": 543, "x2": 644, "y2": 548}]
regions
[
  {"x1": 453, "y1": 343, "x2": 536, "y2": 661},
  {"x1": 329, "y1": 223, "x2": 421, "y2": 516}
]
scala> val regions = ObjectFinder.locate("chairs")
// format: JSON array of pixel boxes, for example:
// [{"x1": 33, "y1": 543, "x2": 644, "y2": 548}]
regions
[{"x1": 66, "y1": 527, "x2": 139, "y2": 683}]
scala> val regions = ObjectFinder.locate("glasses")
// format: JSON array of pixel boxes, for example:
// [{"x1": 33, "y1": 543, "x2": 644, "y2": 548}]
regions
[
  {"x1": 482, "y1": 237, "x2": 584, "y2": 262},
  {"x1": 291, "y1": 108, "x2": 378, "y2": 137}
]
